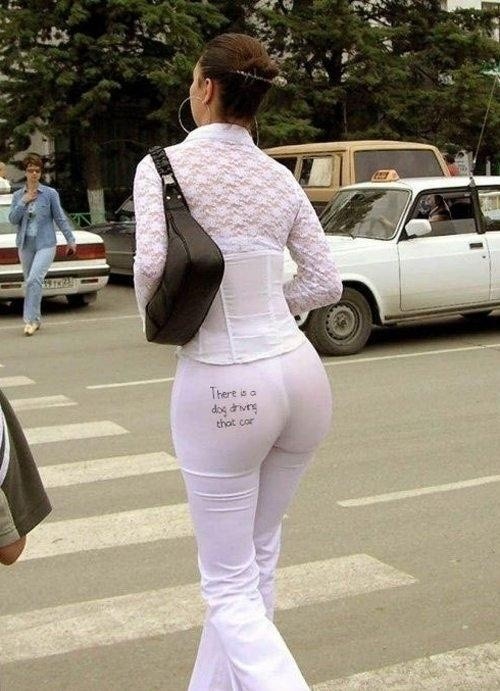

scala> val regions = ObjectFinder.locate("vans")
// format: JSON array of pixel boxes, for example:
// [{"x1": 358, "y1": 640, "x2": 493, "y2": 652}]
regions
[{"x1": 261, "y1": 141, "x2": 452, "y2": 226}]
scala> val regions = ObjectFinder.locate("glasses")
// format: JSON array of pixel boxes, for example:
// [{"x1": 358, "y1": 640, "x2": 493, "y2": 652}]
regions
[{"x1": 27, "y1": 169, "x2": 42, "y2": 174}]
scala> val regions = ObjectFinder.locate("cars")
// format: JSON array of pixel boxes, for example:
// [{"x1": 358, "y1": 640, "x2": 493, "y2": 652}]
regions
[
  {"x1": 82, "y1": 192, "x2": 137, "y2": 276},
  {"x1": 287, "y1": 170, "x2": 499, "y2": 356},
  {"x1": 0, "y1": 178, "x2": 110, "y2": 306}
]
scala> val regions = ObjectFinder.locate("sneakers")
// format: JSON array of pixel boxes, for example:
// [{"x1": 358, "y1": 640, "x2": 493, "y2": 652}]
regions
[{"x1": 24, "y1": 322, "x2": 39, "y2": 336}]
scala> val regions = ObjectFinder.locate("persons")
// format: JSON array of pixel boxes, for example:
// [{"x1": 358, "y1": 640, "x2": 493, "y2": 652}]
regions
[
  {"x1": 442, "y1": 153, "x2": 461, "y2": 177},
  {"x1": 132, "y1": 32, "x2": 345, "y2": 690},
  {"x1": 373, "y1": 193, "x2": 453, "y2": 238},
  {"x1": 7, "y1": 154, "x2": 78, "y2": 337},
  {"x1": 0, "y1": 163, "x2": 11, "y2": 195}
]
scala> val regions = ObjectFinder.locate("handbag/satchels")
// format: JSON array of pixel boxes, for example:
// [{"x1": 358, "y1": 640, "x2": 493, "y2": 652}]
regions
[{"x1": 145, "y1": 199, "x2": 225, "y2": 350}]
